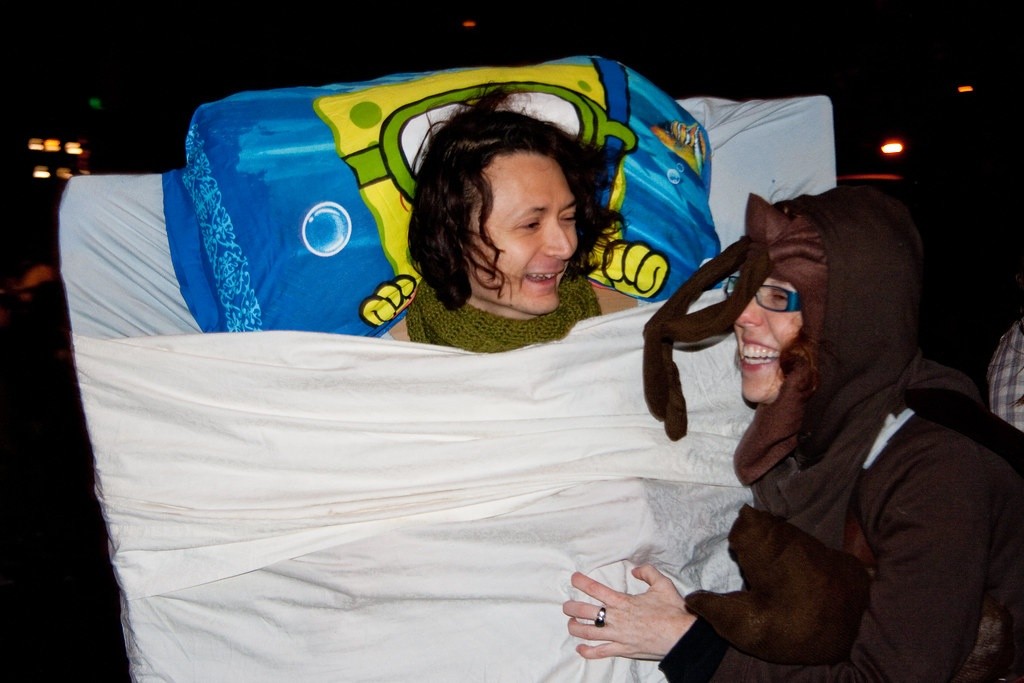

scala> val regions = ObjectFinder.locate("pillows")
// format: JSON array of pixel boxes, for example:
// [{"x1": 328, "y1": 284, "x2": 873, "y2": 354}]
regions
[{"x1": 183, "y1": 56, "x2": 720, "y2": 341}]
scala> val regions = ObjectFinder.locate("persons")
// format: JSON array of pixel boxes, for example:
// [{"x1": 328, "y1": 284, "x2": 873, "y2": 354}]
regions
[
  {"x1": 987, "y1": 313, "x2": 1024, "y2": 432},
  {"x1": 563, "y1": 186, "x2": 1024, "y2": 683},
  {"x1": 403, "y1": 86, "x2": 623, "y2": 356}
]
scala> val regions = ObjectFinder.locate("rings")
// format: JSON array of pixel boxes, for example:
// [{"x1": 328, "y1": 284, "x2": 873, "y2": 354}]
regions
[{"x1": 595, "y1": 607, "x2": 606, "y2": 628}]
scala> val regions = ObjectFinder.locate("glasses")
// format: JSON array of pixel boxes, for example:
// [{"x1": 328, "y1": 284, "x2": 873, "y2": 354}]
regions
[{"x1": 722, "y1": 275, "x2": 802, "y2": 313}]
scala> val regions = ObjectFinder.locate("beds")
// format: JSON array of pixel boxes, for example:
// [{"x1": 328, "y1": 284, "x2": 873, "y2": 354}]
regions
[{"x1": 58, "y1": 95, "x2": 836, "y2": 683}]
